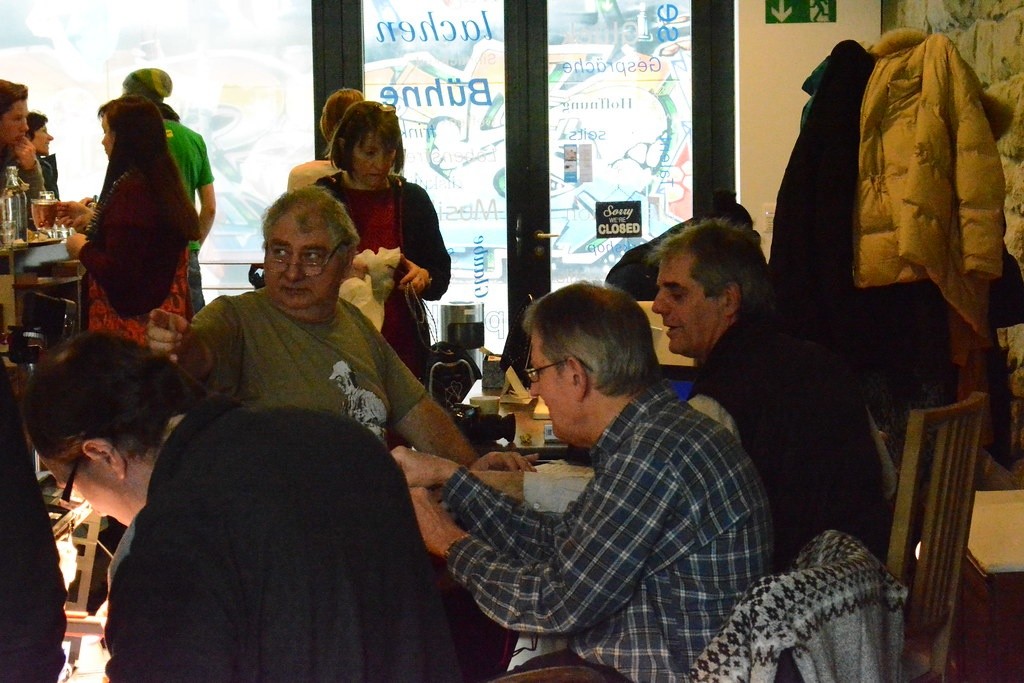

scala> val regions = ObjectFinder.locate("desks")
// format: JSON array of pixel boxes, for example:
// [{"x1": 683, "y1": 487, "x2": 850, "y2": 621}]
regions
[
  {"x1": 0, "y1": 238, "x2": 87, "y2": 366},
  {"x1": 461, "y1": 379, "x2": 569, "y2": 453}
]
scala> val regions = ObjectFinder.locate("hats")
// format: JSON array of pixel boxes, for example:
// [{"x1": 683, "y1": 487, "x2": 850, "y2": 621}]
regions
[{"x1": 124, "y1": 69, "x2": 174, "y2": 103}]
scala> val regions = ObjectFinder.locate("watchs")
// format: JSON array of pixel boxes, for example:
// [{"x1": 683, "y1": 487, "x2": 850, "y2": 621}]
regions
[{"x1": 427, "y1": 272, "x2": 434, "y2": 290}]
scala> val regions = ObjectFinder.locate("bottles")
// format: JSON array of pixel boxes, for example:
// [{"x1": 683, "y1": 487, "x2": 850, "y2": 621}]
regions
[{"x1": 0, "y1": 166, "x2": 28, "y2": 243}]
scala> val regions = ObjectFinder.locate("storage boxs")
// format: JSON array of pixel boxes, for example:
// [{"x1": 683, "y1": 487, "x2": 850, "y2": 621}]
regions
[{"x1": 482, "y1": 355, "x2": 503, "y2": 389}]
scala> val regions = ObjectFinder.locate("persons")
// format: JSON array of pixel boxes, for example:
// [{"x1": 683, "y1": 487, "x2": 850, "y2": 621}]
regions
[
  {"x1": 391, "y1": 281, "x2": 773, "y2": 683},
  {"x1": 0, "y1": 69, "x2": 896, "y2": 683}
]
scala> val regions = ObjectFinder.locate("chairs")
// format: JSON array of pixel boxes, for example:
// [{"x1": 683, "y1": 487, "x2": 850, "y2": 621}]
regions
[{"x1": 886, "y1": 390, "x2": 989, "y2": 683}]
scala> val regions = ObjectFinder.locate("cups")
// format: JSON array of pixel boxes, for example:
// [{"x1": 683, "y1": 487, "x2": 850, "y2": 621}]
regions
[
  {"x1": 30, "y1": 200, "x2": 60, "y2": 236},
  {"x1": 0, "y1": 221, "x2": 17, "y2": 244},
  {"x1": 40, "y1": 191, "x2": 55, "y2": 201},
  {"x1": 470, "y1": 398, "x2": 499, "y2": 417}
]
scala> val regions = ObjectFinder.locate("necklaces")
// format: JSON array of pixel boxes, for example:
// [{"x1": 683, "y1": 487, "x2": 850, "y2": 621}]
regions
[{"x1": 87, "y1": 164, "x2": 134, "y2": 240}]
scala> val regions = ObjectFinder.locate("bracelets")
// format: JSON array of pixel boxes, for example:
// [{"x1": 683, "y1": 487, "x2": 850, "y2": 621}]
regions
[{"x1": 445, "y1": 533, "x2": 469, "y2": 559}]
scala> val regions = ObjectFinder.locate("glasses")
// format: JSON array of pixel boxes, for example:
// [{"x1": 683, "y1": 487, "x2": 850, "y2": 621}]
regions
[
  {"x1": 523, "y1": 355, "x2": 595, "y2": 382},
  {"x1": 264, "y1": 240, "x2": 347, "y2": 278},
  {"x1": 56, "y1": 446, "x2": 88, "y2": 519}
]
